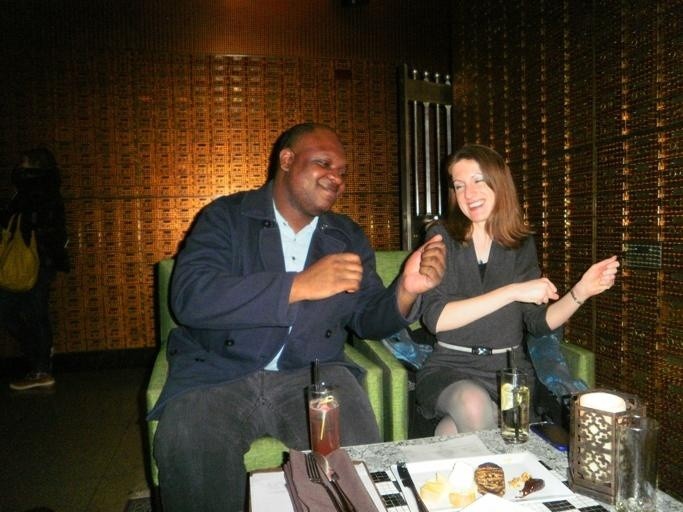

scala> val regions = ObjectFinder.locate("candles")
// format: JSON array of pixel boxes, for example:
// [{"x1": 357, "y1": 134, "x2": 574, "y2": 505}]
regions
[{"x1": 579, "y1": 392, "x2": 626, "y2": 485}]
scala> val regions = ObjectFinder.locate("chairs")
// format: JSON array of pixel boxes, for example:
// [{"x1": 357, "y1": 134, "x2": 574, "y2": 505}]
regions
[
  {"x1": 360, "y1": 251, "x2": 596, "y2": 442},
  {"x1": 146, "y1": 251, "x2": 387, "y2": 489}
]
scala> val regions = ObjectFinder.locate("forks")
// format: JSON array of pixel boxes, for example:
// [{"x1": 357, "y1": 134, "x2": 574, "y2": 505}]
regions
[{"x1": 304, "y1": 454, "x2": 347, "y2": 512}]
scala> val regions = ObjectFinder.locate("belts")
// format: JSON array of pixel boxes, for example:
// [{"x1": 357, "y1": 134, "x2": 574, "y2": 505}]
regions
[{"x1": 437, "y1": 340, "x2": 520, "y2": 357}]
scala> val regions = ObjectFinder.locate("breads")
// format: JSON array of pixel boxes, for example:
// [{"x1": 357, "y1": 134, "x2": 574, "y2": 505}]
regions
[
  {"x1": 509, "y1": 471, "x2": 546, "y2": 498},
  {"x1": 475, "y1": 462, "x2": 505, "y2": 498}
]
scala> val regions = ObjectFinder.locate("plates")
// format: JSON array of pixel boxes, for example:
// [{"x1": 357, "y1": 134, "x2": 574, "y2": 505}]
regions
[{"x1": 390, "y1": 451, "x2": 576, "y2": 511}]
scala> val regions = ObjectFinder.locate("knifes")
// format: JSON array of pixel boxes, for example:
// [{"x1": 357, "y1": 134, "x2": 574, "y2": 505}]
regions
[
  {"x1": 397, "y1": 462, "x2": 428, "y2": 512},
  {"x1": 312, "y1": 453, "x2": 357, "y2": 512}
]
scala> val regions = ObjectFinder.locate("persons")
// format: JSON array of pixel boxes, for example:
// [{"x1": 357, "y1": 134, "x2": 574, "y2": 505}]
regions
[
  {"x1": 143, "y1": 122, "x2": 446, "y2": 510},
  {"x1": 414, "y1": 145, "x2": 621, "y2": 437},
  {"x1": 0, "y1": 146, "x2": 68, "y2": 391}
]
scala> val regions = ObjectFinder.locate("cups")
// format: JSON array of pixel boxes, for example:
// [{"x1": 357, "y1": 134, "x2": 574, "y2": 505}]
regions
[
  {"x1": 501, "y1": 367, "x2": 529, "y2": 444},
  {"x1": 612, "y1": 411, "x2": 661, "y2": 512},
  {"x1": 308, "y1": 382, "x2": 339, "y2": 453}
]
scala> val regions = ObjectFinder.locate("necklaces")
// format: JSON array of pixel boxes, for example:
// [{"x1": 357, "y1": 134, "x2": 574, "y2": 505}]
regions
[{"x1": 478, "y1": 258, "x2": 483, "y2": 264}]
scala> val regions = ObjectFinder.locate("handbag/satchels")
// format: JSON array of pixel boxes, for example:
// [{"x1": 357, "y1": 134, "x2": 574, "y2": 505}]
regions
[{"x1": 1, "y1": 227, "x2": 39, "y2": 291}]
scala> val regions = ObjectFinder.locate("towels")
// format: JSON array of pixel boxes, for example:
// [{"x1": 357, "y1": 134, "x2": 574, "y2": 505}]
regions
[{"x1": 283, "y1": 450, "x2": 379, "y2": 512}]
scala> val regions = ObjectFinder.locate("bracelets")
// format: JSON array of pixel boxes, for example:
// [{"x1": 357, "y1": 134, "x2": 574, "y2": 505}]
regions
[{"x1": 570, "y1": 289, "x2": 584, "y2": 305}]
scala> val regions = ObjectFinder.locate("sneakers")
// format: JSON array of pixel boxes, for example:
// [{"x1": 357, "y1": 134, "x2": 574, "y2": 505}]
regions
[{"x1": 9, "y1": 372, "x2": 54, "y2": 390}]
